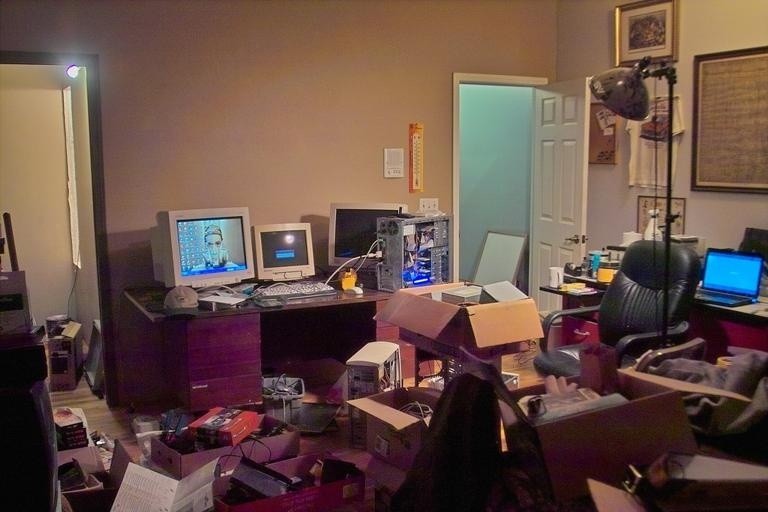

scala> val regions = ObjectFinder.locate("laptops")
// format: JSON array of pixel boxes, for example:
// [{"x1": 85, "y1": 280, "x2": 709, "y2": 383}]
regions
[{"x1": 691, "y1": 247, "x2": 764, "y2": 308}]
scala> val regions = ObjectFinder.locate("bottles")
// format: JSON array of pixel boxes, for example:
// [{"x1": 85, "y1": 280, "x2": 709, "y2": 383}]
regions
[
  {"x1": 592, "y1": 252, "x2": 600, "y2": 278},
  {"x1": 342, "y1": 266, "x2": 355, "y2": 290}
]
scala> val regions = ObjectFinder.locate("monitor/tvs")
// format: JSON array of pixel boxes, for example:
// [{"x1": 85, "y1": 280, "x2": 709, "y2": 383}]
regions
[
  {"x1": 150, "y1": 206, "x2": 255, "y2": 288},
  {"x1": 328, "y1": 201, "x2": 409, "y2": 273},
  {"x1": 254, "y1": 222, "x2": 315, "y2": 281}
]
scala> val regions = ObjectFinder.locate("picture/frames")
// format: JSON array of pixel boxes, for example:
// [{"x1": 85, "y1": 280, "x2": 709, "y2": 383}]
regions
[
  {"x1": 636, "y1": 194, "x2": 686, "y2": 239},
  {"x1": 472, "y1": 229, "x2": 527, "y2": 287},
  {"x1": 587, "y1": 101, "x2": 619, "y2": 166},
  {"x1": 614, "y1": 0, "x2": 679, "y2": 68},
  {"x1": 689, "y1": 44, "x2": 768, "y2": 198}
]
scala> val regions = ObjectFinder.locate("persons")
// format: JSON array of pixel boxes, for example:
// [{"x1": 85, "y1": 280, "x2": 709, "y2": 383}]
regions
[{"x1": 196, "y1": 224, "x2": 237, "y2": 270}]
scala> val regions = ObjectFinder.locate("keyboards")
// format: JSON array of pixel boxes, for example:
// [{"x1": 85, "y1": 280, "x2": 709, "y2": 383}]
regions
[{"x1": 255, "y1": 282, "x2": 337, "y2": 298}]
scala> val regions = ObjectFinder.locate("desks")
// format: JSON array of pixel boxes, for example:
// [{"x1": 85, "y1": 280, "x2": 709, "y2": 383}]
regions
[
  {"x1": 541, "y1": 251, "x2": 768, "y2": 366},
  {"x1": 122, "y1": 267, "x2": 463, "y2": 409}
]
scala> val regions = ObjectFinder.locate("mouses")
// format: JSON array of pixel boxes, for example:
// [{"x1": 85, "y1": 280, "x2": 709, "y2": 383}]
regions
[{"x1": 344, "y1": 287, "x2": 364, "y2": 296}]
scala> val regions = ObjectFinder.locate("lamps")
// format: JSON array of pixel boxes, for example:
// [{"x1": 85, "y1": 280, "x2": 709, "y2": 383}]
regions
[{"x1": 590, "y1": 58, "x2": 678, "y2": 348}]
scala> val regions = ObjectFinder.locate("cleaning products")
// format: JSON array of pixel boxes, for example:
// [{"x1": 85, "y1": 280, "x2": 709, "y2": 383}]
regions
[{"x1": 645, "y1": 209, "x2": 662, "y2": 240}]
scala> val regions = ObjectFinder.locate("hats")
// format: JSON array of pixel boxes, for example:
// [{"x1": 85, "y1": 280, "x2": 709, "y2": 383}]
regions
[{"x1": 162, "y1": 284, "x2": 201, "y2": 317}]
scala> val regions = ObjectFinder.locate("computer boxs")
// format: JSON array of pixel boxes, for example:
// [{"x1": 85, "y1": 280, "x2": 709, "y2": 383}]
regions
[
  {"x1": 345, "y1": 340, "x2": 402, "y2": 453},
  {"x1": 375, "y1": 216, "x2": 451, "y2": 293}
]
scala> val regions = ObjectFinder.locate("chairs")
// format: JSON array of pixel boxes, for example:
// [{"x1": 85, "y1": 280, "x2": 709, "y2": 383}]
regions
[{"x1": 539, "y1": 239, "x2": 703, "y2": 371}]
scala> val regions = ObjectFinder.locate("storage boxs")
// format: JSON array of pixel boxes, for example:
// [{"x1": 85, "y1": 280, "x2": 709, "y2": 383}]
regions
[
  {"x1": 0, "y1": 268, "x2": 139, "y2": 510},
  {"x1": 373, "y1": 280, "x2": 545, "y2": 360},
  {"x1": 503, "y1": 367, "x2": 750, "y2": 498},
  {"x1": 209, "y1": 448, "x2": 367, "y2": 512},
  {"x1": 348, "y1": 383, "x2": 443, "y2": 474},
  {"x1": 584, "y1": 453, "x2": 768, "y2": 511},
  {"x1": 149, "y1": 413, "x2": 303, "y2": 481}
]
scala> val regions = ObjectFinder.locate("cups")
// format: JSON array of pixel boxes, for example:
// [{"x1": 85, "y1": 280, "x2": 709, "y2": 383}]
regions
[
  {"x1": 548, "y1": 267, "x2": 564, "y2": 289},
  {"x1": 597, "y1": 268, "x2": 614, "y2": 282}
]
